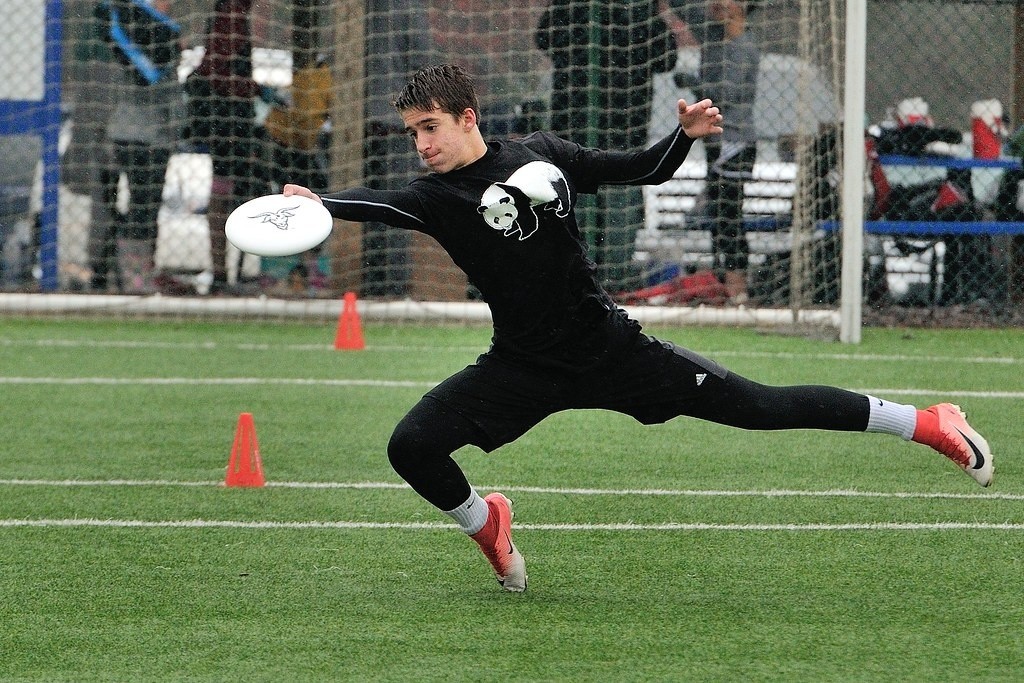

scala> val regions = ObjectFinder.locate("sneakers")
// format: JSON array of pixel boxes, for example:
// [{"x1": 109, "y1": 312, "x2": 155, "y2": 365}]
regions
[
  {"x1": 926, "y1": 402, "x2": 995, "y2": 488},
  {"x1": 470, "y1": 493, "x2": 529, "y2": 593},
  {"x1": 717, "y1": 293, "x2": 751, "y2": 305}
]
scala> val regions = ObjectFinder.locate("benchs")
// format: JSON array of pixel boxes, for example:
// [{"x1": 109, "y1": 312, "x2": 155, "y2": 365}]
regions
[{"x1": 629, "y1": 158, "x2": 946, "y2": 303}]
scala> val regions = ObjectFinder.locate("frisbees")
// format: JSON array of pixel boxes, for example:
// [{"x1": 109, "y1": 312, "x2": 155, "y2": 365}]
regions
[{"x1": 222, "y1": 193, "x2": 336, "y2": 258}]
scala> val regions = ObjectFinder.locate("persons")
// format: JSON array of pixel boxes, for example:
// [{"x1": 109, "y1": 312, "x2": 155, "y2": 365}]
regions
[
  {"x1": 283, "y1": 63, "x2": 993, "y2": 591},
  {"x1": 73, "y1": 1, "x2": 433, "y2": 296},
  {"x1": 533, "y1": 1, "x2": 678, "y2": 292},
  {"x1": 677, "y1": 2, "x2": 760, "y2": 305}
]
